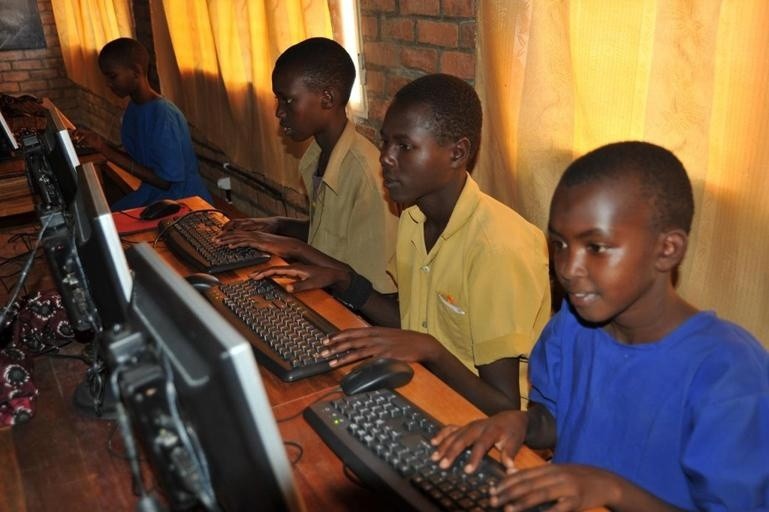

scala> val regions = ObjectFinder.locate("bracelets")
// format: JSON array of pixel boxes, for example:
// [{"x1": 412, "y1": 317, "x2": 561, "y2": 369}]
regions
[{"x1": 330, "y1": 271, "x2": 372, "y2": 310}]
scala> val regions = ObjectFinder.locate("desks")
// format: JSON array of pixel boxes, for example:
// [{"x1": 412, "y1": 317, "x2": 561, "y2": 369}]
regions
[{"x1": 0, "y1": 126, "x2": 610, "y2": 512}]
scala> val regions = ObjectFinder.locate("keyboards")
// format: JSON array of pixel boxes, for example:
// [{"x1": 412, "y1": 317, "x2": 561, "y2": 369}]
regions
[
  {"x1": 160, "y1": 212, "x2": 271, "y2": 272},
  {"x1": 206, "y1": 274, "x2": 348, "y2": 385},
  {"x1": 305, "y1": 387, "x2": 510, "y2": 512},
  {"x1": 73, "y1": 139, "x2": 96, "y2": 156}
]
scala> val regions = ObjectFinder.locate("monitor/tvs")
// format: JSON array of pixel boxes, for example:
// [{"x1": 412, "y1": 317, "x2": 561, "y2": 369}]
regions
[
  {"x1": 42, "y1": 158, "x2": 136, "y2": 412},
  {"x1": 0, "y1": 112, "x2": 19, "y2": 160},
  {"x1": 102, "y1": 242, "x2": 309, "y2": 512},
  {"x1": 20, "y1": 107, "x2": 80, "y2": 209}
]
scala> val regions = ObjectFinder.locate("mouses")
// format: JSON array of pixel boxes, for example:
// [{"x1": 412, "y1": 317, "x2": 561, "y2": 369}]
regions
[
  {"x1": 140, "y1": 198, "x2": 181, "y2": 221},
  {"x1": 186, "y1": 273, "x2": 221, "y2": 292},
  {"x1": 339, "y1": 359, "x2": 416, "y2": 396}
]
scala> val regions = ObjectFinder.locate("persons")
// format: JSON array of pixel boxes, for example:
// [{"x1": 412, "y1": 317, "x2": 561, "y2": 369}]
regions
[
  {"x1": 70, "y1": 38, "x2": 214, "y2": 212},
  {"x1": 211, "y1": 37, "x2": 403, "y2": 303},
  {"x1": 431, "y1": 141, "x2": 769, "y2": 512},
  {"x1": 249, "y1": 72, "x2": 553, "y2": 418}
]
